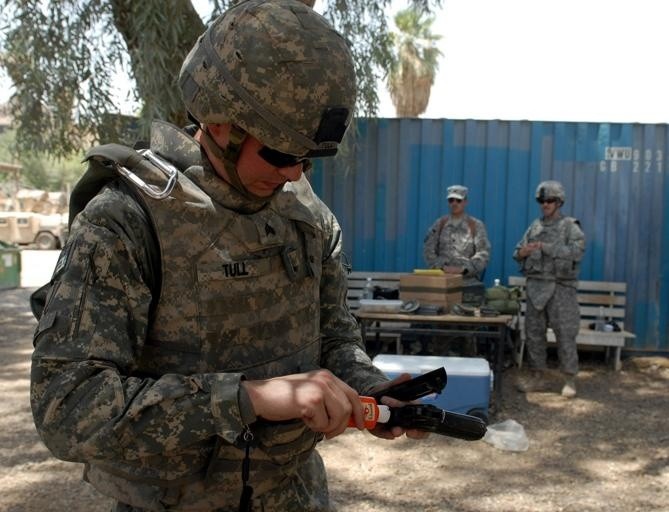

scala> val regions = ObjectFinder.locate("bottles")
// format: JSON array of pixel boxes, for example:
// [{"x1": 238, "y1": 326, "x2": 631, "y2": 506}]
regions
[
  {"x1": 596, "y1": 306, "x2": 605, "y2": 332},
  {"x1": 363, "y1": 278, "x2": 374, "y2": 300},
  {"x1": 347, "y1": 394, "x2": 391, "y2": 428},
  {"x1": 494, "y1": 279, "x2": 500, "y2": 287}
]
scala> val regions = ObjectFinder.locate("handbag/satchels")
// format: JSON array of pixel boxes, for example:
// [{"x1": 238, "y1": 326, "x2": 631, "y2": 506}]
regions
[{"x1": 484, "y1": 285, "x2": 521, "y2": 314}]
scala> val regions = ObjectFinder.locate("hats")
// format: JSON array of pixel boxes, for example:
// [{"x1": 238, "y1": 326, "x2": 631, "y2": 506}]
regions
[{"x1": 447, "y1": 185, "x2": 467, "y2": 199}]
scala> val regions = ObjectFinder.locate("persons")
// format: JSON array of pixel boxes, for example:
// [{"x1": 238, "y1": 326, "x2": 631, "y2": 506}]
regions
[
  {"x1": 424, "y1": 184, "x2": 490, "y2": 357},
  {"x1": 30, "y1": 0, "x2": 431, "y2": 511},
  {"x1": 512, "y1": 180, "x2": 587, "y2": 397}
]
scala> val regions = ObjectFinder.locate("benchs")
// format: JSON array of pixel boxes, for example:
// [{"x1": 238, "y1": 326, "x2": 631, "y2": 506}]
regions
[
  {"x1": 339, "y1": 271, "x2": 420, "y2": 359},
  {"x1": 503, "y1": 274, "x2": 639, "y2": 375}
]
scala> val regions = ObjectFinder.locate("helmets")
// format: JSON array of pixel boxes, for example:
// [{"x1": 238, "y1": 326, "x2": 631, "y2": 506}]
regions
[
  {"x1": 179, "y1": 0, "x2": 355, "y2": 158},
  {"x1": 536, "y1": 181, "x2": 565, "y2": 200}
]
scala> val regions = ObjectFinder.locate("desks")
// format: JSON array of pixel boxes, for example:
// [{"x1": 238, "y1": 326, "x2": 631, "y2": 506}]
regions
[{"x1": 353, "y1": 299, "x2": 515, "y2": 398}]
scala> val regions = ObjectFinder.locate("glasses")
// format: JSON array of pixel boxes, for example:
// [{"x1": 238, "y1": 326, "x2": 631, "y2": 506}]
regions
[{"x1": 257, "y1": 146, "x2": 306, "y2": 168}]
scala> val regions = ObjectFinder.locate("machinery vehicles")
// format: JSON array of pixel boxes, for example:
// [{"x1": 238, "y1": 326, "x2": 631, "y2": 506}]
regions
[{"x1": 0, "y1": 187, "x2": 70, "y2": 248}]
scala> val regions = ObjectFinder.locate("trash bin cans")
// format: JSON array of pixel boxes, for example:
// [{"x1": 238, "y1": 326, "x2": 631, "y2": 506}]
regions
[{"x1": 0, "y1": 240, "x2": 23, "y2": 291}]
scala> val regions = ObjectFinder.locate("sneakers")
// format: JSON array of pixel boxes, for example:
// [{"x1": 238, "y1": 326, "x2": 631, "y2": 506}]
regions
[
  {"x1": 561, "y1": 374, "x2": 578, "y2": 398},
  {"x1": 517, "y1": 371, "x2": 545, "y2": 393}
]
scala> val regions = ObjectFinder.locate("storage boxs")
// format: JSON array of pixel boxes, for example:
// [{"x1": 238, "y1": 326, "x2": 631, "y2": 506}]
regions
[
  {"x1": 363, "y1": 354, "x2": 497, "y2": 427},
  {"x1": 398, "y1": 269, "x2": 464, "y2": 314}
]
